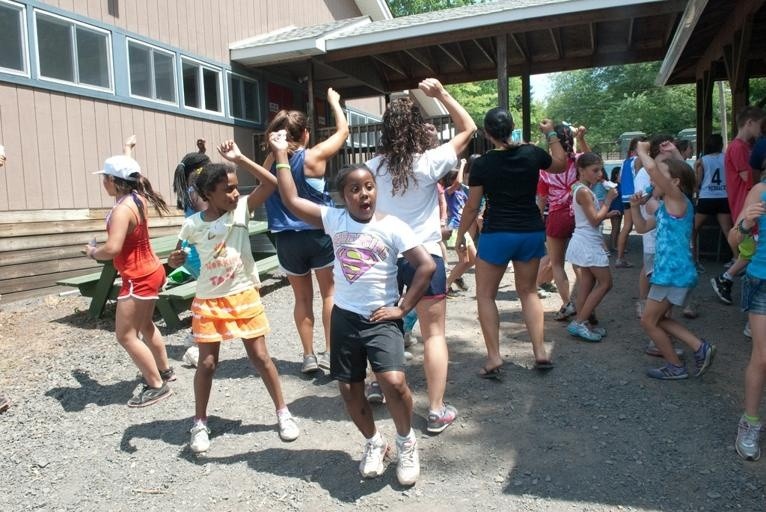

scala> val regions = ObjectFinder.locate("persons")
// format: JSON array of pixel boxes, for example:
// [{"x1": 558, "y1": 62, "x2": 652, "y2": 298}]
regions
[
  {"x1": 160, "y1": 136, "x2": 211, "y2": 303},
  {"x1": 451, "y1": 105, "x2": 566, "y2": 380},
  {"x1": 615, "y1": 132, "x2": 697, "y2": 357},
  {"x1": 743, "y1": 137, "x2": 766, "y2": 343},
  {"x1": 708, "y1": 104, "x2": 765, "y2": 306},
  {"x1": 679, "y1": 132, "x2": 738, "y2": 272},
  {"x1": 357, "y1": 77, "x2": 478, "y2": 436},
  {"x1": 258, "y1": 87, "x2": 351, "y2": 376},
  {"x1": 725, "y1": 135, "x2": 766, "y2": 464},
  {"x1": 537, "y1": 121, "x2": 592, "y2": 319},
  {"x1": 166, "y1": 138, "x2": 301, "y2": 454},
  {"x1": 566, "y1": 155, "x2": 621, "y2": 343},
  {"x1": 632, "y1": 138, "x2": 719, "y2": 381},
  {"x1": 84, "y1": 134, "x2": 179, "y2": 407},
  {"x1": 438, "y1": 136, "x2": 487, "y2": 298},
  {"x1": 264, "y1": 124, "x2": 436, "y2": 486}
]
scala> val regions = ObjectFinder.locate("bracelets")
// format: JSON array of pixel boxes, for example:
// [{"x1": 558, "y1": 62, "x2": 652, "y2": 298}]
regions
[
  {"x1": 546, "y1": 128, "x2": 560, "y2": 145},
  {"x1": 274, "y1": 163, "x2": 291, "y2": 171},
  {"x1": 737, "y1": 218, "x2": 753, "y2": 234},
  {"x1": 87, "y1": 247, "x2": 98, "y2": 261}
]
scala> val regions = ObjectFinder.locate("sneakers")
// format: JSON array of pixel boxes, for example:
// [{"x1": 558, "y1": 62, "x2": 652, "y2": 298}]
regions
[
  {"x1": 635, "y1": 272, "x2": 753, "y2": 381},
  {"x1": 127, "y1": 363, "x2": 177, "y2": 409},
  {"x1": 443, "y1": 271, "x2": 469, "y2": 297},
  {"x1": 426, "y1": 405, "x2": 458, "y2": 434},
  {"x1": 535, "y1": 281, "x2": 608, "y2": 341},
  {"x1": 366, "y1": 381, "x2": 386, "y2": 405},
  {"x1": 278, "y1": 411, "x2": 301, "y2": 441},
  {"x1": 300, "y1": 350, "x2": 331, "y2": 374},
  {"x1": 606, "y1": 246, "x2": 635, "y2": 269},
  {"x1": 402, "y1": 335, "x2": 418, "y2": 360},
  {"x1": 734, "y1": 414, "x2": 764, "y2": 462},
  {"x1": 190, "y1": 421, "x2": 211, "y2": 452},
  {"x1": 358, "y1": 427, "x2": 422, "y2": 488}
]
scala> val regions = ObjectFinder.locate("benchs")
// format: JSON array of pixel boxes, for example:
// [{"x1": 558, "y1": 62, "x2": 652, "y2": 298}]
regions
[
  {"x1": 158, "y1": 254, "x2": 285, "y2": 332},
  {"x1": 56, "y1": 256, "x2": 172, "y2": 319}
]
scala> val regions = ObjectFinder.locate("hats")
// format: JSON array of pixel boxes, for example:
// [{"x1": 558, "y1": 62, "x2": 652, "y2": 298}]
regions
[{"x1": 91, "y1": 154, "x2": 143, "y2": 183}]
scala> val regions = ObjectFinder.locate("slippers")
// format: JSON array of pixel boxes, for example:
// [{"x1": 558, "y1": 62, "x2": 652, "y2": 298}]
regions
[
  {"x1": 477, "y1": 365, "x2": 503, "y2": 380},
  {"x1": 534, "y1": 359, "x2": 555, "y2": 371}
]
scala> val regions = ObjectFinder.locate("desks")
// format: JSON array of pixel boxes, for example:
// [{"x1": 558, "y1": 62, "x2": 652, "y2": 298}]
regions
[{"x1": 150, "y1": 220, "x2": 272, "y2": 265}]
